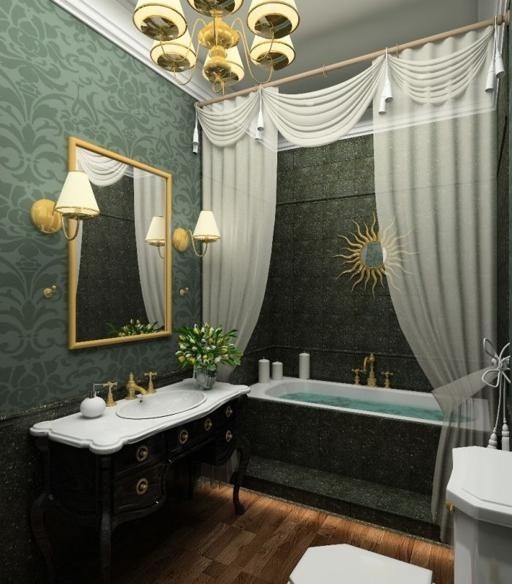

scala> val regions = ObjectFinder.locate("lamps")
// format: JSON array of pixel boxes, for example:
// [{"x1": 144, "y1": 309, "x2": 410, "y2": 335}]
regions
[
  {"x1": 174, "y1": 209, "x2": 219, "y2": 257},
  {"x1": 31, "y1": 171, "x2": 100, "y2": 239},
  {"x1": 133, "y1": 0, "x2": 300, "y2": 95},
  {"x1": 145, "y1": 216, "x2": 167, "y2": 259}
]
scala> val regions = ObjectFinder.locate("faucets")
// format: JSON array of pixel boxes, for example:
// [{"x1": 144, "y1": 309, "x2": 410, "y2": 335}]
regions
[
  {"x1": 126, "y1": 371, "x2": 147, "y2": 400},
  {"x1": 363, "y1": 353, "x2": 376, "y2": 378}
]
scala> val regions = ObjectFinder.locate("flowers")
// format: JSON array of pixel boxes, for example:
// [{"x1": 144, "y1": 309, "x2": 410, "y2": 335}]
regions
[
  {"x1": 174, "y1": 322, "x2": 241, "y2": 370},
  {"x1": 105, "y1": 318, "x2": 164, "y2": 336}
]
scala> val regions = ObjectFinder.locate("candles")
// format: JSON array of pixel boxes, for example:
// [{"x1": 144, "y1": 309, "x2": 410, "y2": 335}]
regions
[
  {"x1": 258, "y1": 355, "x2": 269, "y2": 382},
  {"x1": 298, "y1": 350, "x2": 310, "y2": 378},
  {"x1": 273, "y1": 359, "x2": 283, "y2": 379}
]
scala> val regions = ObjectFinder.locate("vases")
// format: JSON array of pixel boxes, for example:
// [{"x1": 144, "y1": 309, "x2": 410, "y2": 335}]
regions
[{"x1": 193, "y1": 367, "x2": 217, "y2": 388}]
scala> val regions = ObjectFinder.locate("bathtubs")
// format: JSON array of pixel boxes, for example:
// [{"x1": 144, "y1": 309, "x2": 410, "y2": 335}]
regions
[{"x1": 247, "y1": 375, "x2": 491, "y2": 433}]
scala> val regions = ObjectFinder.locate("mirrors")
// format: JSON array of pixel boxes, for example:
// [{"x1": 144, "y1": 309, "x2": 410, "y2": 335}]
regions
[
  {"x1": 68, "y1": 135, "x2": 174, "y2": 349},
  {"x1": 332, "y1": 210, "x2": 420, "y2": 299}
]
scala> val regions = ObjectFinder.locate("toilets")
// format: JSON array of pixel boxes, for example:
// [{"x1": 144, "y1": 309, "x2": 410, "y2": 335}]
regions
[{"x1": 286, "y1": 446, "x2": 512, "y2": 584}]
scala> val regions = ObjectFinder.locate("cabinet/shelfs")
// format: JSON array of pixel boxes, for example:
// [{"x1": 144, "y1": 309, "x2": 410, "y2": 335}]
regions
[{"x1": 29, "y1": 393, "x2": 265, "y2": 584}]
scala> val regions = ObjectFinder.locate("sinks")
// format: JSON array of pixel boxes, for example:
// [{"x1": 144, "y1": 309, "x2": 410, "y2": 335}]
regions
[{"x1": 115, "y1": 389, "x2": 206, "y2": 420}]
